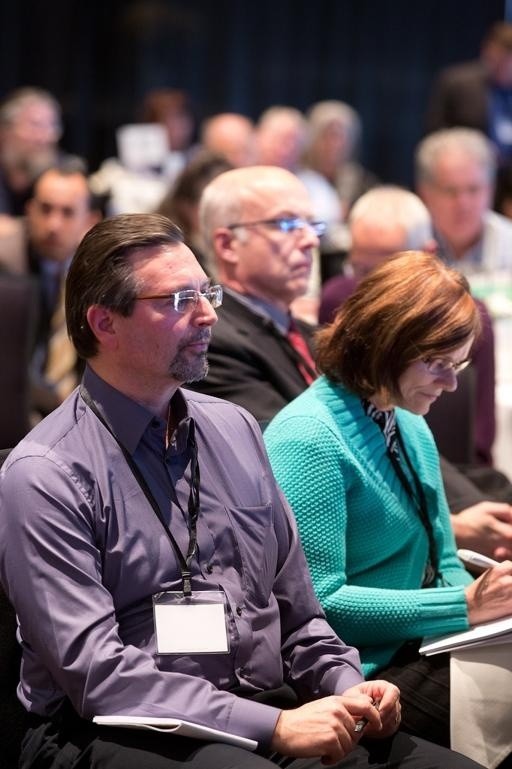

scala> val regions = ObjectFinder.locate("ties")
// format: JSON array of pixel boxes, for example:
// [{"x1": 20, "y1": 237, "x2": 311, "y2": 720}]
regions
[{"x1": 286, "y1": 319, "x2": 324, "y2": 386}]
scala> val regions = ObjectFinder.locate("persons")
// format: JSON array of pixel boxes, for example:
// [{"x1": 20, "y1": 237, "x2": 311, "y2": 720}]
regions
[
  {"x1": 0, "y1": 21, "x2": 511, "y2": 561},
  {"x1": 1, "y1": 215, "x2": 485, "y2": 768},
  {"x1": 262, "y1": 249, "x2": 511, "y2": 769}
]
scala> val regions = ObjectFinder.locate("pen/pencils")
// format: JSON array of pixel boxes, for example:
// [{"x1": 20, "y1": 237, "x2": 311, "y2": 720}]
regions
[
  {"x1": 456, "y1": 548, "x2": 499, "y2": 568},
  {"x1": 354, "y1": 697, "x2": 382, "y2": 732}
]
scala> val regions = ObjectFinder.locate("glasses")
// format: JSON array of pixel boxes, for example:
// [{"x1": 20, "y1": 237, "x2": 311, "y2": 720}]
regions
[
  {"x1": 407, "y1": 336, "x2": 471, "y2": 376},
  {"x1": 227, "y1": 216, "x2": 327, "y2": 237},
  {"x1": 128, "y1": 285, "x2": 224, "y2": 314}
]
